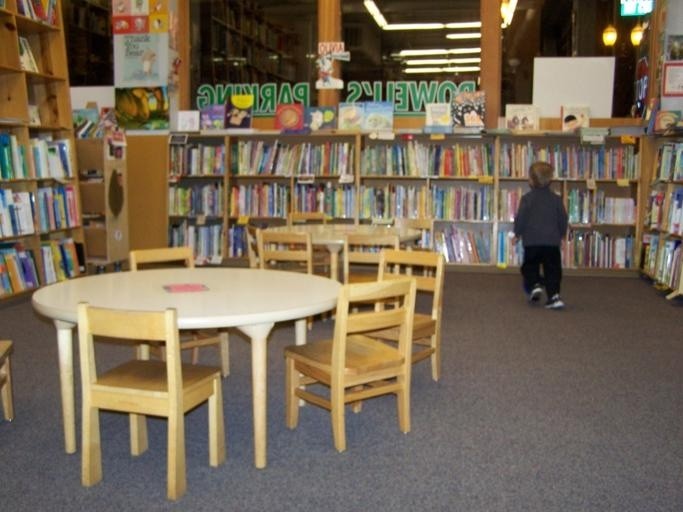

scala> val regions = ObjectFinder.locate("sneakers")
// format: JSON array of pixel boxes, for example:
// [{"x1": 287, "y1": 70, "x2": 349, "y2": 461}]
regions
[
  {"x1": 529, "y1": 283, "x2": 543, "y2": 303},
  {"x1": 544, "y1": 294, "x2": 564, "y2": 309}
]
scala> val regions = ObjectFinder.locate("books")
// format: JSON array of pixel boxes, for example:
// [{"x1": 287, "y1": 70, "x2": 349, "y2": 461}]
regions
[
  {"x1": 640, "y1": 95, "x2": 682, "y2": 288},
  {"x1": 363, "y1": 91, "x2": 492, "y2": 265},
  {"x1": 199, "y1": 2, "x2": 312, "y2": 84},
  {"x1": 499, "y1": 105, "x2": 640, "y2": 270},
  {"x1": 168, "y1": 93, "x2": 363, "y2": 267},
  {"x1": 0, "y1": 0, "x2": 125, "y2": 297}
]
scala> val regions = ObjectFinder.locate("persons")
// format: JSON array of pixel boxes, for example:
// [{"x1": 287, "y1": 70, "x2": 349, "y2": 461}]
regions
[{"x1": 508, "y1": 159, "x2": 569, "y2": 309}]
